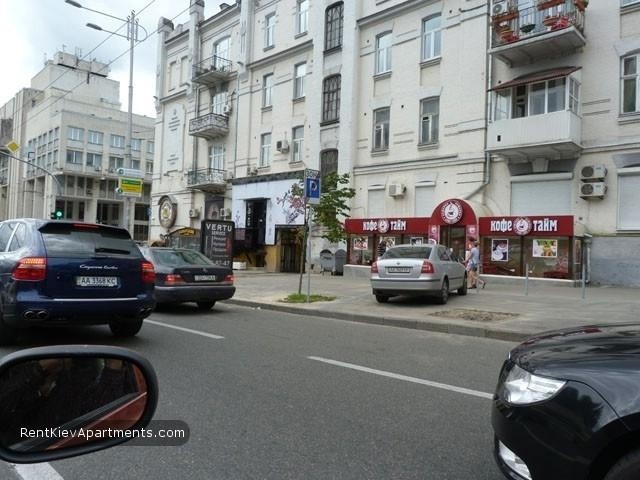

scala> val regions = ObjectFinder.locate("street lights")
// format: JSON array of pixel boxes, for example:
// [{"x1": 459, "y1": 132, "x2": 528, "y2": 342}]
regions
[{"x1": 65, "y1": 0, "x2": 148, "y2": 236}]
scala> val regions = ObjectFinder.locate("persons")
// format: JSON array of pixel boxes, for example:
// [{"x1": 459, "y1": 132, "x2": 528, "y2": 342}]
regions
[{"x1": 462, "y1": 235, "x2": 485, "y2": 289}]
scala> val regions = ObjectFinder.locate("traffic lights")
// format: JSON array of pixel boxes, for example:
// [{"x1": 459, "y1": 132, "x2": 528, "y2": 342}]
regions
[{"x1": 55, "y1": 210, "x2": 63, "y2": 217}]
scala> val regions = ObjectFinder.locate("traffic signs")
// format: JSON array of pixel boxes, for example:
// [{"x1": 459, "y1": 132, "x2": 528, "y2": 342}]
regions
[
  {"x1": 116, "y1": 178, "x2": 142, "y2": 198},
  {"x1": 117, "y1": 168, "x2": 145, "y2": 179}
]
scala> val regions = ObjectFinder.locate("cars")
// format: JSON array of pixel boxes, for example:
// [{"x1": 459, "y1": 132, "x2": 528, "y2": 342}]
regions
[
  {"x1": 1, "y1": 218, "x2": 157, "y2": 346},
  {"x1": 0, "y1": 343, "x2": 158, "y2": 463},
  {"x1": 370, "y1": 243, "x2": 468, "y2": 304},
  {"x1": 491, "y1": 322, "x2": 640, "y2": 479},
  {"x1": 139, "y1": 246, "x2": 236, "y2": 313}
]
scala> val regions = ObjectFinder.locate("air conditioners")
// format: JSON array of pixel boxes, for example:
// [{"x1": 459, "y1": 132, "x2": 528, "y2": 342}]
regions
[
  {"x1": 276, "y1": 141, "x2": 289, "y2": 153},
  {"x1": 491, "y1": 2, "x2": 510, "y2": 18},
  {"x1": 576, "y1": 164, "x2": 607, "y2": 200},
  {"x1": 388, "y1": 183, "x2": 403, "y2": 198}
]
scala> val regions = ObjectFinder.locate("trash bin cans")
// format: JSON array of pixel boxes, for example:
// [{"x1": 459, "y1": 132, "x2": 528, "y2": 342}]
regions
[{"x1": 319, "y1": 247, "x2": 347, "y2": 276}]
